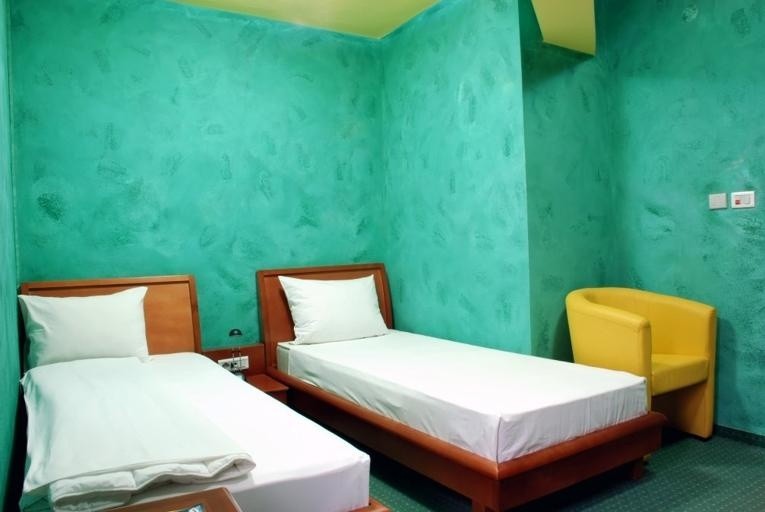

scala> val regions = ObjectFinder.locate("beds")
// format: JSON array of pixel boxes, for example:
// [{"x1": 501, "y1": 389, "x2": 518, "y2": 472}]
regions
[
  {"x1": 14, "y1": 274, "x2": 394, "y2": 511},
  {"x1": 253, "y1": 262, "x2": 670, "y2": 511}
]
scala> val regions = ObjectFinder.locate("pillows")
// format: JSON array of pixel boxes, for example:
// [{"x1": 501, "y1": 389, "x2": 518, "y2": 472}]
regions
[
  {"x1": 14, "y1": 285, "x2": 152, "y2": 372},
  {"x1": 276, "y1": 272, "x2": 395, "y2": 348}
]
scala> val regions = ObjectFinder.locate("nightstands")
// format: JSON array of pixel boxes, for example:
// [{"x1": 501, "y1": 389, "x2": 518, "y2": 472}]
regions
[{"x1": 202, "y1": 343, "x2": 292, "y2": 407}]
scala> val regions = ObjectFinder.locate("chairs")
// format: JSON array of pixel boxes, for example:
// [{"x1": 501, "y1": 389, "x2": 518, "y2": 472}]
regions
[{"x1": 562, "y1": 281, "x2": 721, "y2": 452}]
